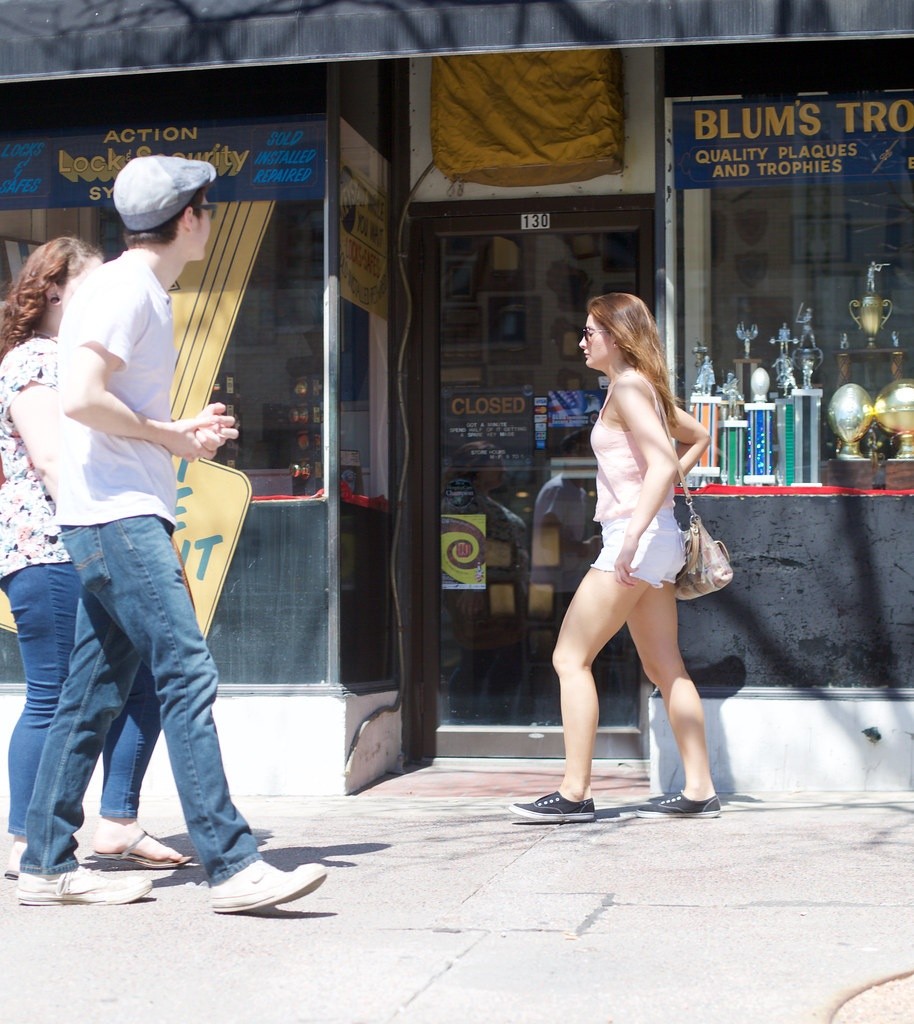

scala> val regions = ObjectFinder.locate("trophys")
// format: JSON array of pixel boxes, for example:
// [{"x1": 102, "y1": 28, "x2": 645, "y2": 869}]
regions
[
  {"x1": 688, "y1": 355, "x2": 721, "y2": 477},
  {"x1": 744, "y1": 368, "x2": 776, "y2": 485},
  {"x1": 832, "y1": 262, "x2": 908, "y2": 390},
  {"x1": 719, "y1": 373, "x2": 749, "y2": 485},
  {"x1": 770, "y1": 303, "x2": 824, "y2": 487},
  {"x1": 733, "y1": 321, "x2": 763, "y2": 394}
]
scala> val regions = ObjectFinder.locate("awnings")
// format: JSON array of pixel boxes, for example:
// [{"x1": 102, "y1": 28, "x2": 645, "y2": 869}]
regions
[{"x1": 0, "y1": 0, "x2": 914, "y2": 82}]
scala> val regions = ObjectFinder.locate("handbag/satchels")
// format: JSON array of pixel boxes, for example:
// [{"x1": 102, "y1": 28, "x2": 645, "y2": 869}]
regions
[{"x1": 673, "y1": 517, "x2": 733, "y2": 601}]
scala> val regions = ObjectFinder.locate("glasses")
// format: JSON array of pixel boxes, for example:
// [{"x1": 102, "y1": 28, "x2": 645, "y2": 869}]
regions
[
  {"x1": 193, "y1": 203, "x2": 217, "y2": 220},
  {"x1": 582, "y1": 326, "x2": 608, "y2": 342}
]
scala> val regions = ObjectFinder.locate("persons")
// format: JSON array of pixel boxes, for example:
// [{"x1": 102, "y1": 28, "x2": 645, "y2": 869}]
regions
[
  {"x1": 510, "y1": 292, "x2": 722, "y2": 824},
  {"x1": 530, "y1": 426, "x2": 601, "y2": 595},
  {"x1": 15, "y1": 155, "x2": 325, "y2": 912},
  {"x1": 443, "y1": 439, "x2": 530, "y2": 726},
  {"x1": 0, "y1": 235, "x2": 193, "y2": 879}
]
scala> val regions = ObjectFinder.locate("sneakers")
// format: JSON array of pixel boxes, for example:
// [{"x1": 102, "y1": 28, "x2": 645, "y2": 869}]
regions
[
  {"x1": 210, "y1": 861, "x2": 327, "y2": 915},
  {"x1": 509, "y1": 791, "x2": 596, "y2": 821},
  {"x1": 636, "y1": 790, "x2": 721, "y2": 818},
  {"x1": 16, "y1": 861, "x2": 151, "y2": 906}
]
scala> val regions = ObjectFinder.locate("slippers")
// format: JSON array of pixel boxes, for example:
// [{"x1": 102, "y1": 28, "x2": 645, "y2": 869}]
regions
[
  {"x1": 95, "y1": 832, "x2": 191, "y2": 868},
  {"x1": 5, "y1": 871, "x2": 19, "y2": 879}
]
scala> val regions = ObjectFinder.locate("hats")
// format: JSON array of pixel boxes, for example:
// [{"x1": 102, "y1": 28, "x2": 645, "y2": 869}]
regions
[{"x1": 113, "y1": 156, "x2": 216, "y2": 231}]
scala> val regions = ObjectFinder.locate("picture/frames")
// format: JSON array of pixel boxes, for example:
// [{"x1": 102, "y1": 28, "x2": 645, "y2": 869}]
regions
[{"x1": 434, "y1": 234, "x2": 544, "y2": 389}]
